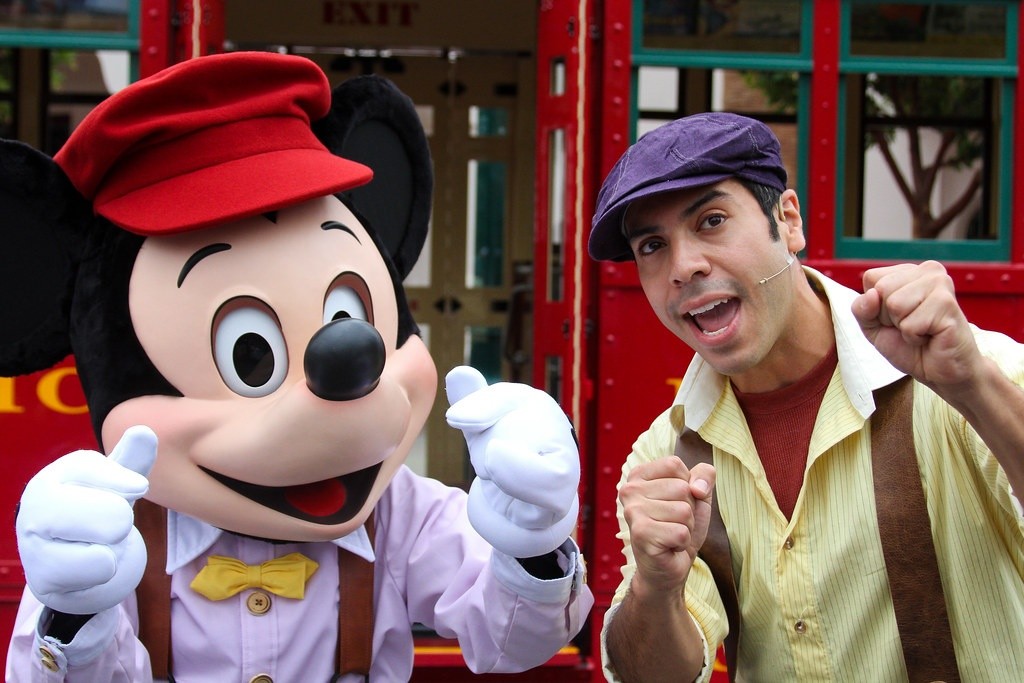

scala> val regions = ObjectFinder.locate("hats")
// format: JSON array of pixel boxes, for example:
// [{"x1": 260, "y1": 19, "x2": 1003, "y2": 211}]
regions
[{"x1": 587, "y1": 111, "x2": 788, "y2": 265}]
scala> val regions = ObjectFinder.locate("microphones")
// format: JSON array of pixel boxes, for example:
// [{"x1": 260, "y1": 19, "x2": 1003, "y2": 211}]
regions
[{"x1": 760, "y1": 257, "x2": 794, "y2": 285}]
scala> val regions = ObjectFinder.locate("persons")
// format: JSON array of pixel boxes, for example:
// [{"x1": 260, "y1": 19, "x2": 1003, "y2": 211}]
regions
[
  {"x1": 579, "y1": 110, "x2": 1024, "y2": 683},
  {"x1": 0, "y1": 50, "x2": 599, "y2": 683}
]
